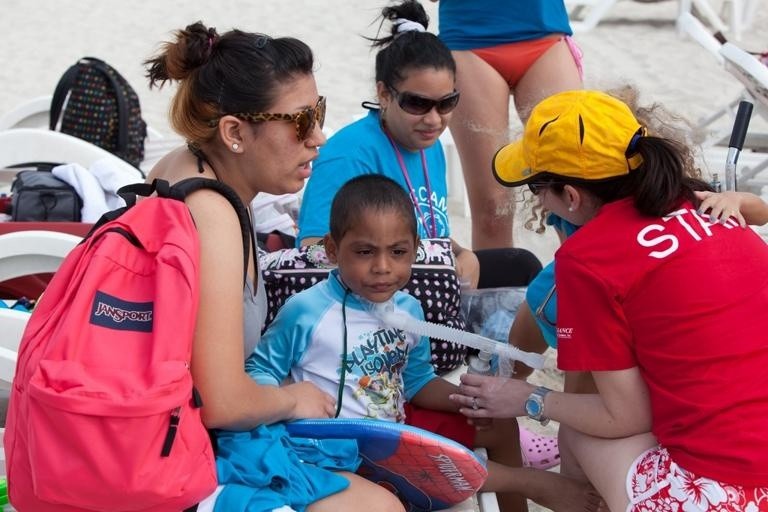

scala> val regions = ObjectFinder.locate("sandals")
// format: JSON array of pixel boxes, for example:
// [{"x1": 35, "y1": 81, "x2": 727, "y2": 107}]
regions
[{"x1": 519, "y1": 423, "x2": 561, "y2": 471}]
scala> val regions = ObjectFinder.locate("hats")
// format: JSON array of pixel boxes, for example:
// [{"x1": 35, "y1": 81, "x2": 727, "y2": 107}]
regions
[{"x1": 492, "y1": 90, "x2": 647, "y2": 189}]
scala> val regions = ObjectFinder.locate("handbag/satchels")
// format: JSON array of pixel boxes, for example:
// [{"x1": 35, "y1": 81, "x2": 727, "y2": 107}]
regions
[{"x1": 9, "y1": 171, "x2": 83, "y2": 222}]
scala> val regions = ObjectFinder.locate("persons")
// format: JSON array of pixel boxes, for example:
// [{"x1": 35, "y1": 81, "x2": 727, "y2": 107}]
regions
[
  {"x1": 505, "y1": 86, "x2": 768, "y2": 383},
  {"x1": 134, "y1": 21, "x2": 406, "y2": 512},
  {"x1": 295, "y1": 0, "x2": 559, "y2": 469},
  {"x1": 430, "y1": 0, "x2": 584, "y2": 250},
  {"x1": 449, "y1": 91, "x2": 768, "y2": 511},
  {"x1": 245, "y1": 173, "x2": 605, "y2": 512}
]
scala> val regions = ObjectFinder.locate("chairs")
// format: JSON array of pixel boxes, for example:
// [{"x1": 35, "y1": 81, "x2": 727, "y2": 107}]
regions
[
  {"x1": 0, "y1": 94, "x2": 162, "y2": 146},
  {"x1": 0, "y1": 307, "x2": 504, "y2": 512},
  {"x1": 0, "y1": 230, "x2": 85, "y2": 282},
  {"x1": 2, "y1": 118, "x2": 146, "y2": 220}
]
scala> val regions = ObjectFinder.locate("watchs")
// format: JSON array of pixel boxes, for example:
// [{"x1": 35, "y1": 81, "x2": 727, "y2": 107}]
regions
[{"x1": 524, "y1": 387, "x2": 553, "y2": 421}]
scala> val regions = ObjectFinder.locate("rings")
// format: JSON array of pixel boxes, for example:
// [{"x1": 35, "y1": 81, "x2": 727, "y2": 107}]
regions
[{"x1": 471, "y1": 397, "x2": 478, "y2": 410}]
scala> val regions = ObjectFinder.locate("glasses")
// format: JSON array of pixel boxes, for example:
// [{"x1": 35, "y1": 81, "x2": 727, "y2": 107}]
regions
[
  {"x1": 384, "y1": 78, "x2": 461, "y2": 116},
  {"x1": 207, "y1": 95, "x2": 326, "y2": 144},
  {"x1": 525, "y1": 178, "x2": 576, "y2": 195}
]
